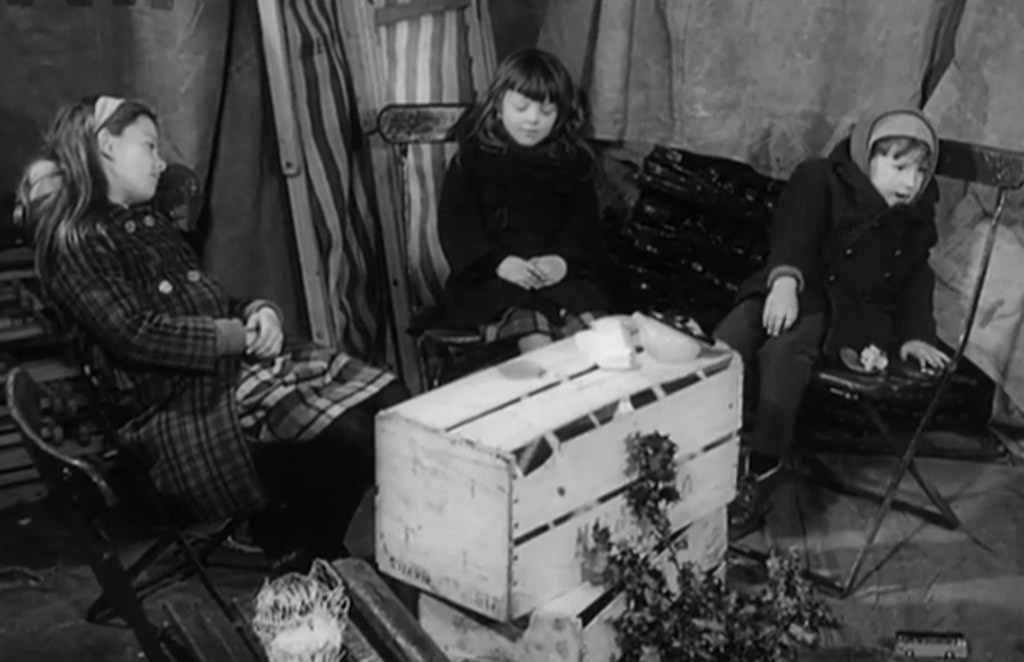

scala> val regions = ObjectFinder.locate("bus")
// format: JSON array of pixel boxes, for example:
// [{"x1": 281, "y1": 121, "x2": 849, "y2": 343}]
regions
[{"x1": 895, "y1": 631, "x2": 970, "y2": 661}]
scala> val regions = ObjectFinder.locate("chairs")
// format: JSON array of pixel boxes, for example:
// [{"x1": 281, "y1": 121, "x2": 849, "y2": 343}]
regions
[
  {"x1": 725, "y1": 138, "x2": 1024, "y2": 600},
  {"x1": 7, "y1": 165, "x2": 309, "y2": 660},
  {"x1": 378, "y1": 100, "x2": 498, "y2": 393}
]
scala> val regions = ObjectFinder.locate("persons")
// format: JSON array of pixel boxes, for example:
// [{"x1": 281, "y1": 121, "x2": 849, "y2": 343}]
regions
[
  {"x1": 711, "y1": 106, "x2": 951, "y2": 541},
  {"x1": 30, "y1": 95, "x2": 410, "y2": 615},
  {"x1": 405, "y1": 49, "x2": 621, "y2": 353}
]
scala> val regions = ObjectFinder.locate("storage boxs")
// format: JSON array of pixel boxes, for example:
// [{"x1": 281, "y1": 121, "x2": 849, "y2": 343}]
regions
[
  {"x1": 374, "y1": 313, "x2": 743, "y2": 620},
  {"x1": 418, "y1": 505, "x2": 729, "y2": 661}
]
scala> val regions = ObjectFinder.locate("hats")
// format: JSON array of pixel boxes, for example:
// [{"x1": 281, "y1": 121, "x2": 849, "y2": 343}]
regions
[{"x1": 868, "y1": 114, "x2": 935, "y2": 160}]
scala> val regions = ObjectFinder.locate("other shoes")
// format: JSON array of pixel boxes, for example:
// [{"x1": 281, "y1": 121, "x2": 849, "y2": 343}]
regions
[{"x1": 726, "y1": 454, "x2": 783, "y2": 538}]
